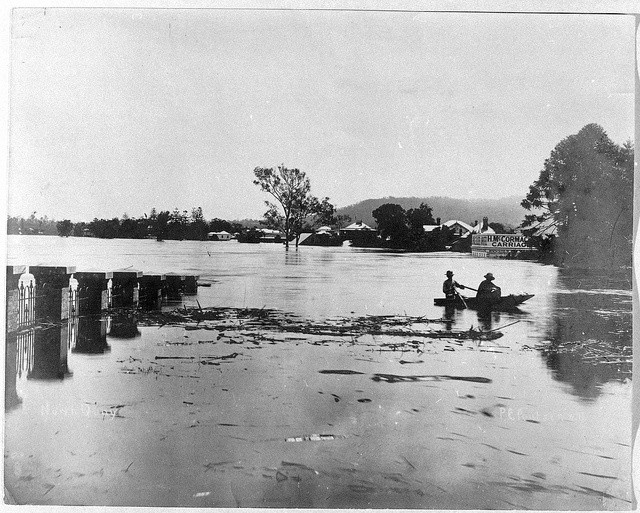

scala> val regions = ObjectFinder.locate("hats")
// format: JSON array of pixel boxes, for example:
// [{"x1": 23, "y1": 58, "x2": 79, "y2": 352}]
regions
[
  {"x1": 445, "y1": 271, "x2": 454, "y2": 275},
  {"x1": 484, "y1": 273, "x2": 495, "y2": 280}
]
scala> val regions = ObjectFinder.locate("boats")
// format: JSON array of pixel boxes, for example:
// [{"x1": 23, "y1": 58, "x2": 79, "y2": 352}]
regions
[{"x1": 433, "y1": 293, "x2": 536, "y2": 306}]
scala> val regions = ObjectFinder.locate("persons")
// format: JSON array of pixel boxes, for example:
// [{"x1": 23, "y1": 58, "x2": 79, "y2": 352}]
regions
[
  {"x1": 443, "y1": 271, "x2": 464, "y2": 300},
  {"x1": 476, "y1": 273, "x2": 501, "y2": 298}
]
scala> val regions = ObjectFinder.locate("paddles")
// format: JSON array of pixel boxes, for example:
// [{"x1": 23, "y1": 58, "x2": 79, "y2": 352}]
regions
[{"x1": 452, "y1": 287, "x2": 469, "y2": 309}]
